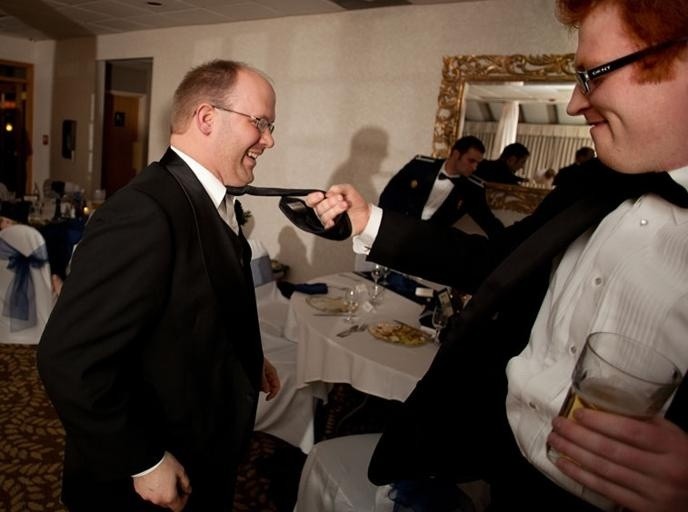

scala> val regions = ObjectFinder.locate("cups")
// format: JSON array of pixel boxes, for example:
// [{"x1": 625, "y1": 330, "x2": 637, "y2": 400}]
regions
[{"x1": 542, "y1": 329, "x2": 684, "y2": 495}]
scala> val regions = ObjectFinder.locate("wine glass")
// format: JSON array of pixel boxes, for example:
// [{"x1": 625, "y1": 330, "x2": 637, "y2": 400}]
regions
[
  {"x1": 341, "y1": 287, "x2": 361, "y2": 322},
  {"x1": 431, "y1": 303, "x2": 450, "y2": 347},
  {"x1": 366, "y1": 262, "x2": 392, "y2": 320}
]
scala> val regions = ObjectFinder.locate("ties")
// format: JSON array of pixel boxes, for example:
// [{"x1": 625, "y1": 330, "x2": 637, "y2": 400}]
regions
[{"x1": 226, "y1": 185, "x2": 351, "y2": 241}]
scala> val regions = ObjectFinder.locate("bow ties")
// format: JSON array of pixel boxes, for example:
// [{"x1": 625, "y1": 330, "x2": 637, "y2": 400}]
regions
[
  {"x1": 601, "y1": 171, "x2": 687, "y2": 209},
  {"x1": 439, "y1": 172, "x2": 460, "y2": 185}
]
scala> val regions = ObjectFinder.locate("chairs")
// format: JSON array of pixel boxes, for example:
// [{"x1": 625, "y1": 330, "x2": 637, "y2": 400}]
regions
[
  {"x1": 295, "y1": 434, "x2": 379, "y2": 506},
  {"x1": 0, "y1": 225, "x2": 59, "y2": 345},
  {"x1": 250, "y1": 238, "x2": 394, "y2": 453}
]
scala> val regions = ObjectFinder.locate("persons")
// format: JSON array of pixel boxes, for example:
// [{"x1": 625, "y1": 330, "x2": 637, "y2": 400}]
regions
[
  {"x1": 367, "y1": 136, "x2": 505, "y2": 283},
  {"x1": 480, "y1": 142, "x2": 530, "y2": 186},
  {"x1": 36, "y1": 58, "x2": 352, "y2": 512},
  {"x1": 554, "y1": 147, "x2": 596, "y2": 189},
  {"x1": 303, "y1": 0, "x2": 687, "y2": 511}
]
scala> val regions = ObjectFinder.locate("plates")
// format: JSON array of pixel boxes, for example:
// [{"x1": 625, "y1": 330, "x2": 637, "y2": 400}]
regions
[{"x1": 365, "y1": 318, "x2": 437, "y2": 348}]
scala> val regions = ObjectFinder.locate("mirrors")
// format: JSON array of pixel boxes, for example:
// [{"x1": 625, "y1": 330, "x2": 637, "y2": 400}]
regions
[{"x1": 431, "y1": 53, "x2": 596, "y2": 216}]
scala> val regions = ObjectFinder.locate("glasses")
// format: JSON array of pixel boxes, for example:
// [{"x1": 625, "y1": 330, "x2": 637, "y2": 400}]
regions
[
  {"x1": 575, "y1": 32, "x2": 688, "y2": 96},
  {"x1": 192, "y1": 105, "x2": 274, "y2": 135}
]
